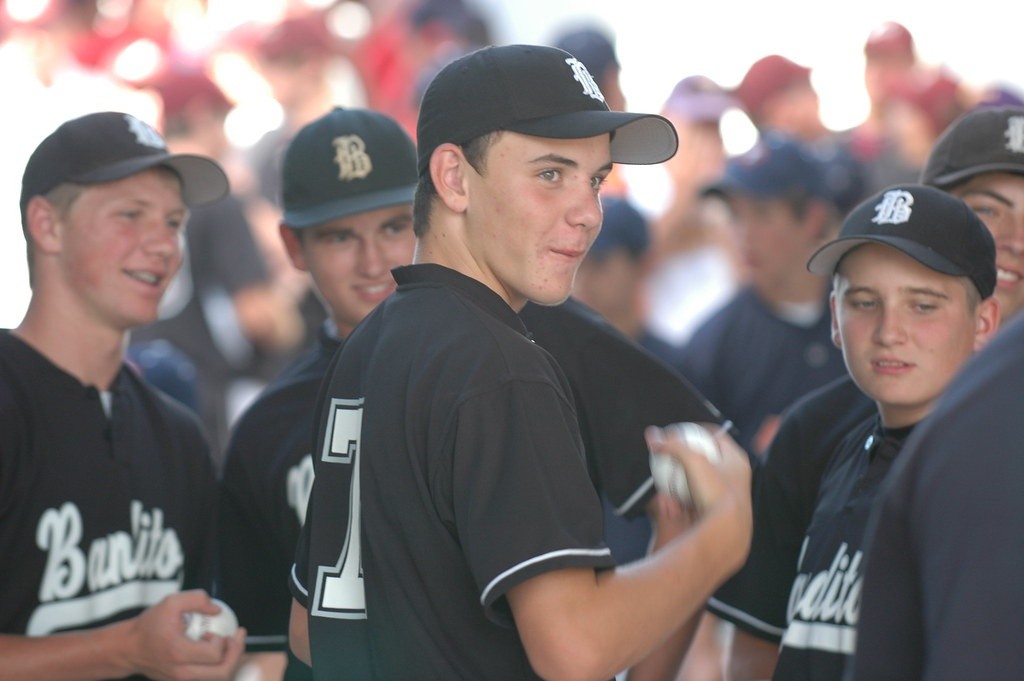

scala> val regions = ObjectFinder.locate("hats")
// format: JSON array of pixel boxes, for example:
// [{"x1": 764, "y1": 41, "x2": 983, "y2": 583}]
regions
[
  {"x1": 281, "y1": 108, "x2": 419, "y2": 229},
  {"x1": 417, "y1": 44, "x2": 678, "y2": 176},
  {"x1": 918, "y1": 106, "x2": 1024, "y2": 186},
  {"x1": 697, "y1": 132, "x2": 873, "y2": 211},
  {"x1": 809, "y1": 183, "x2": 997, "y2": 300},
  {"x1": 22, "y1": 112, "x2": 229, "y2": 207},
  {"x1": 586, "y1": 195, "x2": 647, "y2": 253}
]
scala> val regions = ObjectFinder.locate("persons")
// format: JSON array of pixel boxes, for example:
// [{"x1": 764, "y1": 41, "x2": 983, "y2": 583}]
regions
[
  {"x1": 769, "y1": 185, "x2": 1002, "y2": 681},
  {"x1": 284, "y1": 44, "x2": 755, "y2": 681},
  {"x1": 551, "y1": 22, "x2": 1024, "y2": 681},
  {"x1": 121, "y1": 0, "x2": 498, "y2": 681},
  {"x1": 0, "y1": 113, "x2": 248, "y2": 681}
]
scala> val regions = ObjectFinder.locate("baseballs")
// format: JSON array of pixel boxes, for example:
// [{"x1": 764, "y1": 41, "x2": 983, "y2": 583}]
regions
[
  {"x1": 649, "y1": 420, "x2": 723, "y2": 511},
  {"x1": 184, "y1": 596, "x2": 239, "y2": 642}
]
